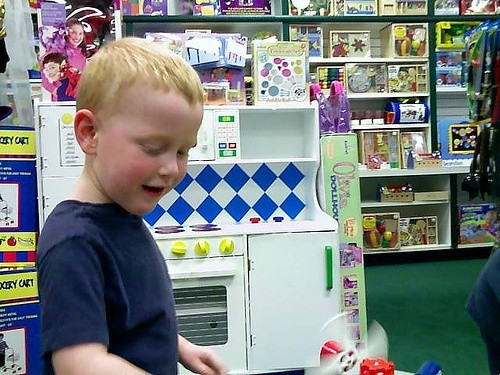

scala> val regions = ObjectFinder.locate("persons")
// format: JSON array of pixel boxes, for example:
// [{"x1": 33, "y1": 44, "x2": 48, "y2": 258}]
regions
[
  {"x1": 38, "y1": 18, "x2": 86, "y2": 103},
  {"x1": 36, "y1": 33, "x2": 228, "y2": 375},
  {"x1": 41, "y1": 51, "x2": 81, "y2": 102}
]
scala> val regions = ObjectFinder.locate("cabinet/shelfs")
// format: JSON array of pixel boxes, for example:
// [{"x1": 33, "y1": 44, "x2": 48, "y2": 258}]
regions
[
  {"x1": 246, "y1": 231, "x2": 341, "y2": 375},
  {"x1": 120, "y1": 0, "x2": 500, "y2": 255}
]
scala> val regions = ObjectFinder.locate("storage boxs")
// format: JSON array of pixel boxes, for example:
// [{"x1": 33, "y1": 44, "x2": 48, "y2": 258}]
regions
[
  {"x1": 316, "y1": 134, "x2": 368, "y2": 343},
  {"x1": 121, "y1": 0, "x2": 500, "y2": 251}
]
